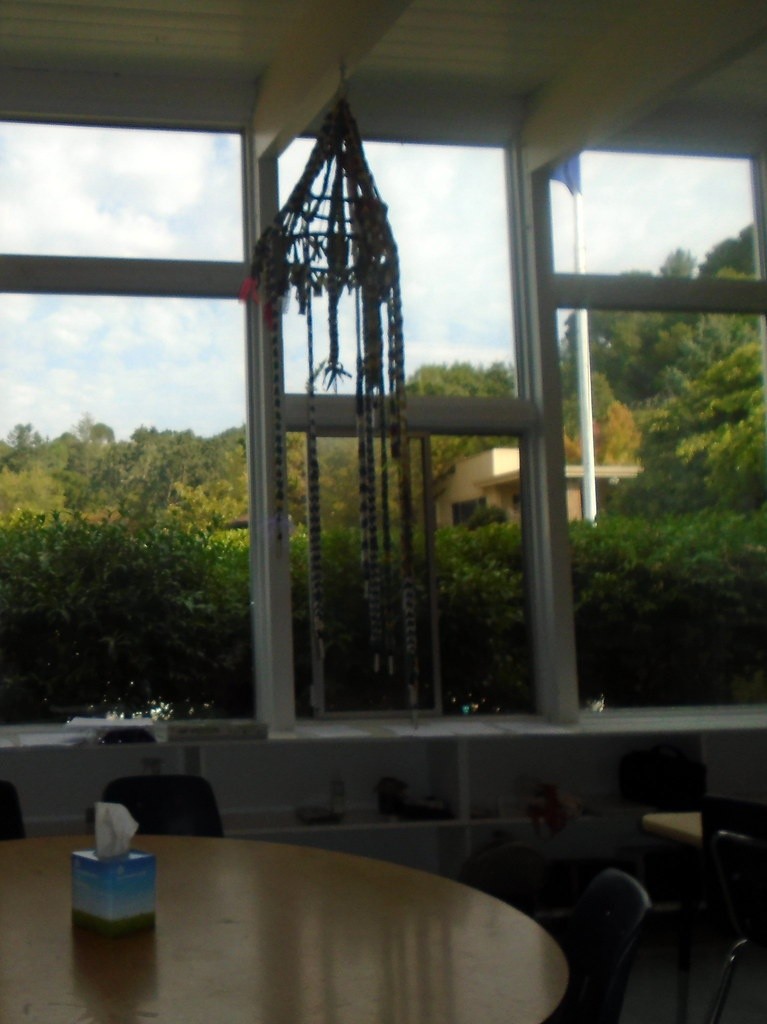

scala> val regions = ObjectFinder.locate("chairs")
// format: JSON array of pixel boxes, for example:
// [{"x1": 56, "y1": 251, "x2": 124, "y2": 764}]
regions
[
  {"x1": 558, "y1": 869, "x2": 653, "y2": 1024},
  {"x1": 698, "y1": 795, "x2": 767, "y2": 1024},
  {"x1": 102, "y1": 774, "x2": 225, "y2": 839}
]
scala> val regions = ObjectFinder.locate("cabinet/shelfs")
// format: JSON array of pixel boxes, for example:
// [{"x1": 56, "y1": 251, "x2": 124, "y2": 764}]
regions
[{"x1": 0, "y1": 706, "x2": 767, "y2": 925}]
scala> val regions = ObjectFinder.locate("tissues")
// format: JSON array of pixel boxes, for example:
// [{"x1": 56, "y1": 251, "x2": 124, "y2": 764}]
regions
[{"x1": 70, "y1": 800, "x2": 156, "y2": 947}]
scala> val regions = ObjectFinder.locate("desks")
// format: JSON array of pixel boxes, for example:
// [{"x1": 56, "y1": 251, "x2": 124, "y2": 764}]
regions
[
  {"x1": 640, "y1": 810, "x2": 702, "y2": 1024},
  {"x1": 0, "y1": 833, "x2": 571, "y2": 1023}
]
